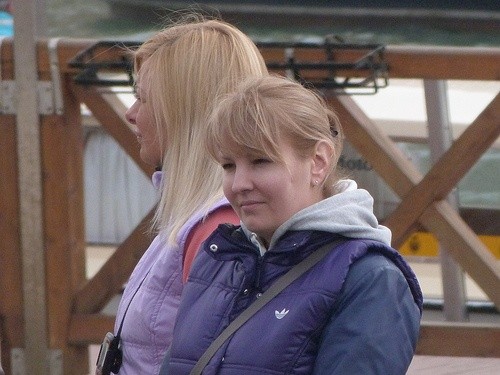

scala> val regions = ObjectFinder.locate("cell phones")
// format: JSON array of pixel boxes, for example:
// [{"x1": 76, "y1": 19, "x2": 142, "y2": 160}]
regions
[{"x1": 95, "y1": 332, "x2": 117, "y2": 375}]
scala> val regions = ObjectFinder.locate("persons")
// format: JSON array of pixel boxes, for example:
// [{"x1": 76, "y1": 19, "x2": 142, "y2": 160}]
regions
[
  {"x1": 158, "y1": 74, "x2": 422, "y2": 375},
  {"x1": 116, "y1": 13, "x2": 269, "y2": 375}
]
